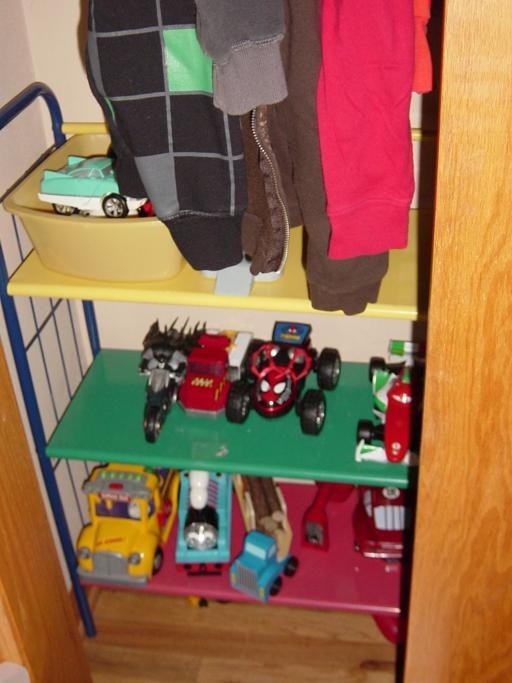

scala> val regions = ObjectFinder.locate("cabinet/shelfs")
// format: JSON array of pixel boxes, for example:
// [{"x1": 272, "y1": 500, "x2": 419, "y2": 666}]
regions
[{"x1": 1, "y1": 82, "x2": 440, "y2": 638}]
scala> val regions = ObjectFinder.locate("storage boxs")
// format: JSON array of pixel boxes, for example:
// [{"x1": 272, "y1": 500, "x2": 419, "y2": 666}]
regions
[{"x1": 2, "y1": 132, "x2": 187, "y2": 283}]
